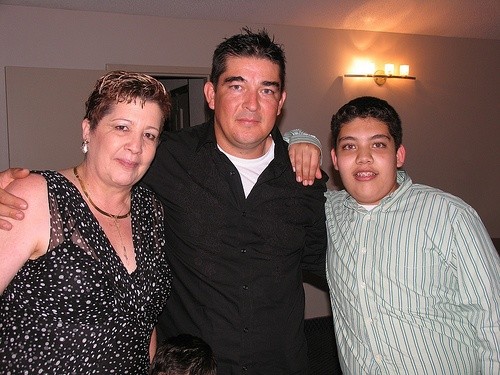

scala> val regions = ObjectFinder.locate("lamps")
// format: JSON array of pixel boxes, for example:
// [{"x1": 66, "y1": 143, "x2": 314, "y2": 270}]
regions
[{"x1": 344, "y1": 64, "x2": 416, "y2": 86}]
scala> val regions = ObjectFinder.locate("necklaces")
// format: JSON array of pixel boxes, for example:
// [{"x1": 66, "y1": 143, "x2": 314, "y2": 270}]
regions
[{"x1": 73, "y1": 166, "x2": 132, "y2": 268}]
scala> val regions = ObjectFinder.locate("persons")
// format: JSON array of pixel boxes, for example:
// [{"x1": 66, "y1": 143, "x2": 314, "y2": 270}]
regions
[
  {"x1": 282, "y1": 96, "x2": 500, "y2": 375},
  {"x1": 0, "y1": 69, "x2": 173, "y2": 375},
  {"x1": 0, "y1": 29, "x2": 330, "y2": 375},
  {"x1": 149, "y1": 333, "x2": 217, "y2": 375}
]
